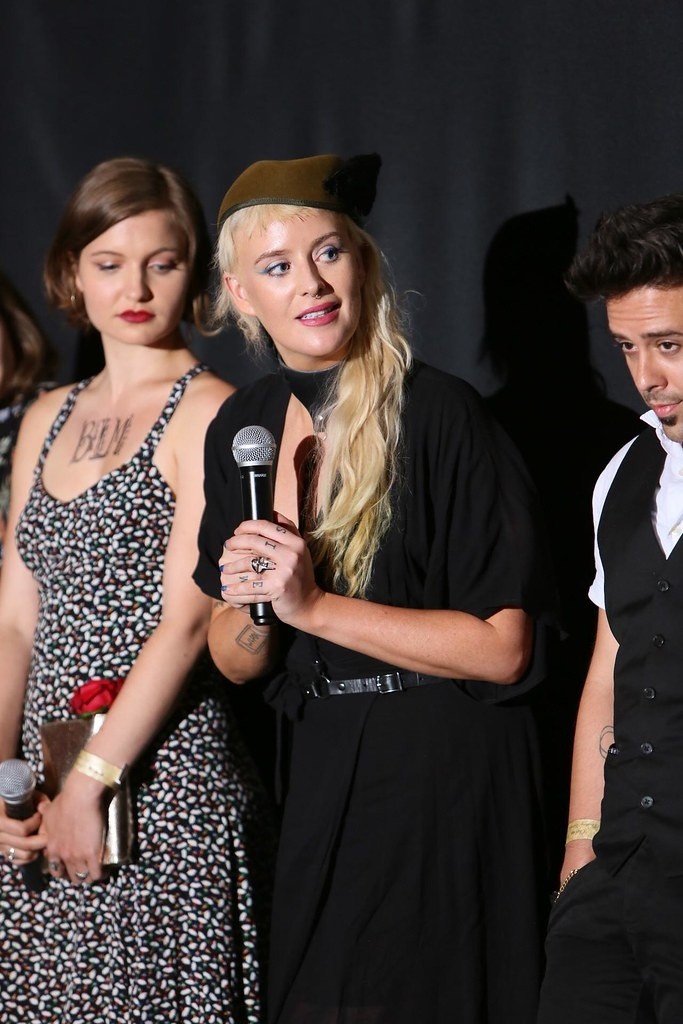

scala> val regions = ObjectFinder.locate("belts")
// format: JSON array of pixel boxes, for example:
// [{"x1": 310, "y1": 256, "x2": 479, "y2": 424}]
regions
[{"x1": 301, "y1": 672, "x2": 450, "y2": 698}]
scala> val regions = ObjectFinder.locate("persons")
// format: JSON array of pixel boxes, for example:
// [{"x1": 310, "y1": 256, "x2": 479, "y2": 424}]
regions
[
  {"x1": 0, "y1": 274, "x2": 60, "y2": 532},
  {"x1": 195, "y1": 153, "x2": 560, "y2": 1022},
  {"x1": 0, "y1": 156, "x2": 263, "y2": 1022},
  {"x1": 537, "y1": 193, "x2": 682, "y2": 1024}
]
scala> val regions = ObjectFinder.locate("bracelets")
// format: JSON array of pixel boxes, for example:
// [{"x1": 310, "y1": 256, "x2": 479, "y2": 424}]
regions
[
  {"x1": 252, "y1": 556, "x2": 276, "y2": 574},
  {"x1": 566, "y1": 819, "x2": 600, "y2": 844},
  {"x1": 75, "y1": 750, "x2": 126, "y2": 790}
]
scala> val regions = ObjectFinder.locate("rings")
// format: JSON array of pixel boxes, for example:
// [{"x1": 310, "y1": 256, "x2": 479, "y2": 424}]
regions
[
  {"x1": 9, "y1": 847, "x2": 15, "y2": 860},
  {"x1": 75, "y1": 871, "x2": 89, "y2": 879},
  {"x1": 48, "y1": 860, "x2": 61, "y2": 870}
]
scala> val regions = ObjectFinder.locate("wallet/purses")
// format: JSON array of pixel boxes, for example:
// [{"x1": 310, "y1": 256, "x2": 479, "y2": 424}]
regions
[{"x1": 39, "y1": 714, "x2": 133, "y2": 867}]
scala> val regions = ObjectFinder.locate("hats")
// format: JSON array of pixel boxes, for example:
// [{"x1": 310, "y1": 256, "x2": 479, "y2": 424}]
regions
[{"x1": 216, "y1": 155, "x2": 356, "y2": 232}]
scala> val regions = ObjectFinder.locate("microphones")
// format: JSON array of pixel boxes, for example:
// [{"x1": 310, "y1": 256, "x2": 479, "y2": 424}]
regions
[
  {"x1": 231, "y1": 425, "x2": 277, "y2": 626},
  {"x1": 0, "y1": 759, "x2": 48, "y2": 891}
]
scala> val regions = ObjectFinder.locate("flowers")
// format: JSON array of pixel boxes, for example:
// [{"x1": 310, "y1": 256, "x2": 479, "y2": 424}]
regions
[{"x1": 68, "y1": 679, "x2": 125, "y2": 720}]
